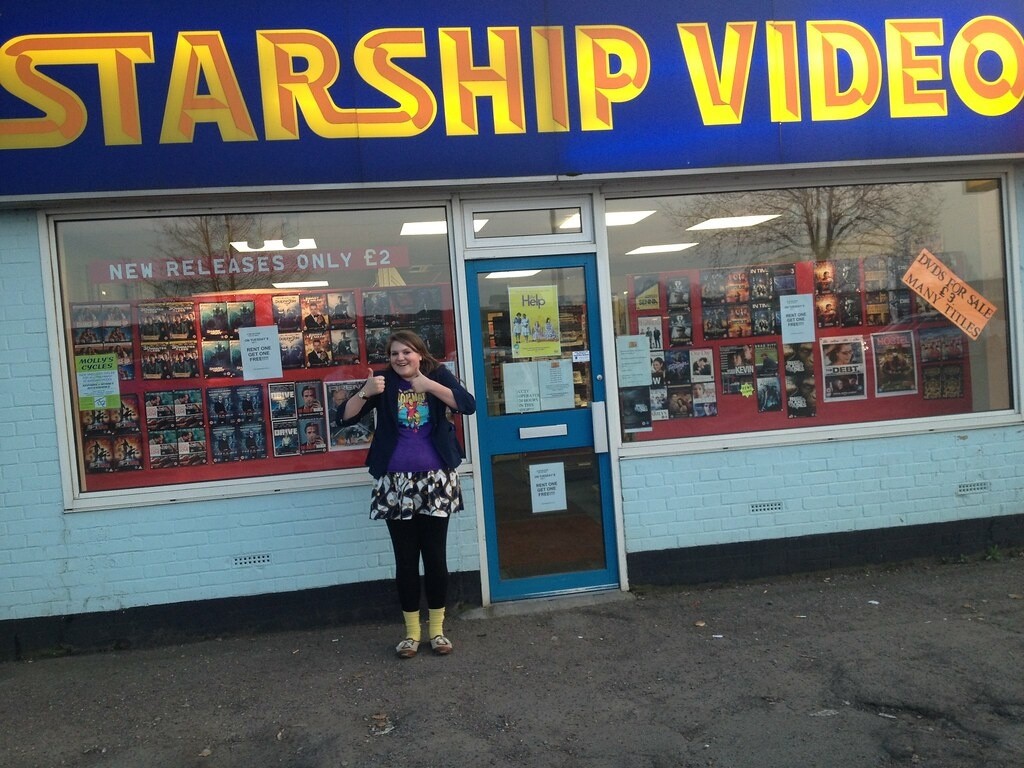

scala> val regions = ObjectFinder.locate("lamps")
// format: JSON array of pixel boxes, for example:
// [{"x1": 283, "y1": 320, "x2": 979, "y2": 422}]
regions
[
  {"x1": 246, "y1": 221, "x2": 265, "y2": 249},
  {"x1": 280, "y1": 218, "x2": 300, "y2": 247}
]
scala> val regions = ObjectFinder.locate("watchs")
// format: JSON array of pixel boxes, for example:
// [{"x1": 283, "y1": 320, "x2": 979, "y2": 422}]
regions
[{"x1": 357, "y1": 391, "x2": 372, "y2": 401}]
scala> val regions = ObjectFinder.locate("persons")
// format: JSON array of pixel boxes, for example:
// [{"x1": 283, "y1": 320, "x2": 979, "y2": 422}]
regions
[
  {"x1": 147, "y1": 391, "x2": 205, "y2": 468},
  {"x1": 73, "y1": 306, "x2": 134, "y2": 380},
  {"x1": 514, "y1": 313, "x2": 557, "y2": 343},
  {"x1": 639, "y1": 280, "x2": 692, "y2": 347},
  {"x1": 332, "y1": 330, "x2": 475, "y2": 661},
  {"x1": 729, "y1": 345, "x2": 778, "y2": 411},
  {"x1": 815, "y1": 271, "x2": 836, "y2": 317},
  {"x1": 921, "y1": 336, "x2": 962, "y2": 360},
  {"x1": 329, "y1": 390, "x2": 349, "y2": 426},
  {"x1": 650, "y1": 357, "x2": 717, "y2": 419},
  {"x1": 787, "y1": 342, "x2": 816, "y2": 416},
  {"x1": 277, "y1": 296, "x2": 355, "y2": 369},
  {"x1": 272, "y1": 386, "x2": 325, "y2": 454},
  {"x1": 823, "y1": 344, "x2": 863, "y2": 397},
  {"x1": 214, "y1": 390, "x2": 258, "y2": 461}
]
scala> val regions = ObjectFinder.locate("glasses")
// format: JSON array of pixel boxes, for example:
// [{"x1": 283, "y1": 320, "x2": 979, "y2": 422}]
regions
[{"x1": 842, "y1": 350, "x2": 854, "y2": 355}]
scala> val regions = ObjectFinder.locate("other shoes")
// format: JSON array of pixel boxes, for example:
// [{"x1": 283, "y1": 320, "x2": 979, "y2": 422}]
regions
[
  {"x1": 430, "y1": 635, "x2": 453, "y2": 656},
  {"x1": 395, "y1": 638, "x2": 420, "y2": 659}
]
